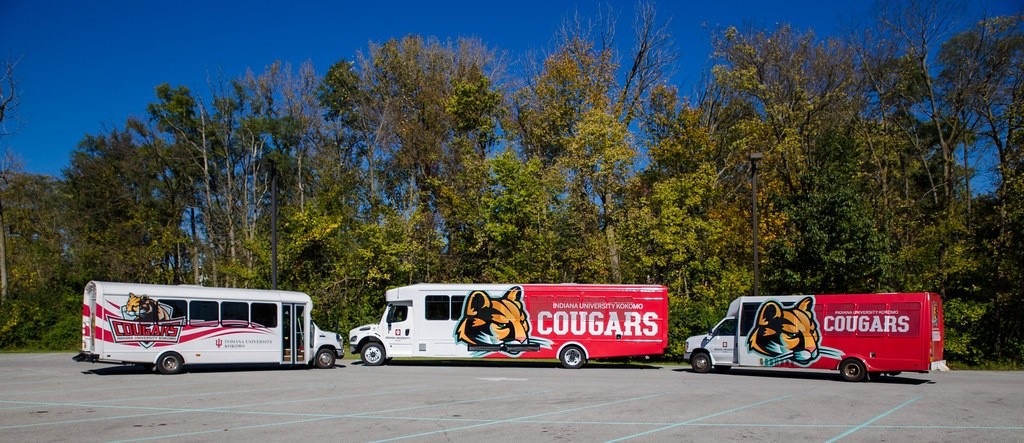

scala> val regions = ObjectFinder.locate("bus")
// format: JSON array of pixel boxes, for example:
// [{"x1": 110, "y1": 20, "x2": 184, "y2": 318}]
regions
[
  {"x1": 81, "y1": 279, "x2": 345, "y2": 375},
  {"x1": 348, "y1": 281, "x2": 670, "y2": 370},
  {"x1": 683, "y1": 292, "x2": 951, "y2": 382}
]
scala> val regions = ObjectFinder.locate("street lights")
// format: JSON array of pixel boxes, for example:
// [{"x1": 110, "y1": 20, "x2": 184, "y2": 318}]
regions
[
  {"x1": 265, "y1": 156, "x2": 281, "y2": 293},
  {"x1": 748, "y1": 152, "x2": 763, "y2": 296}
]
